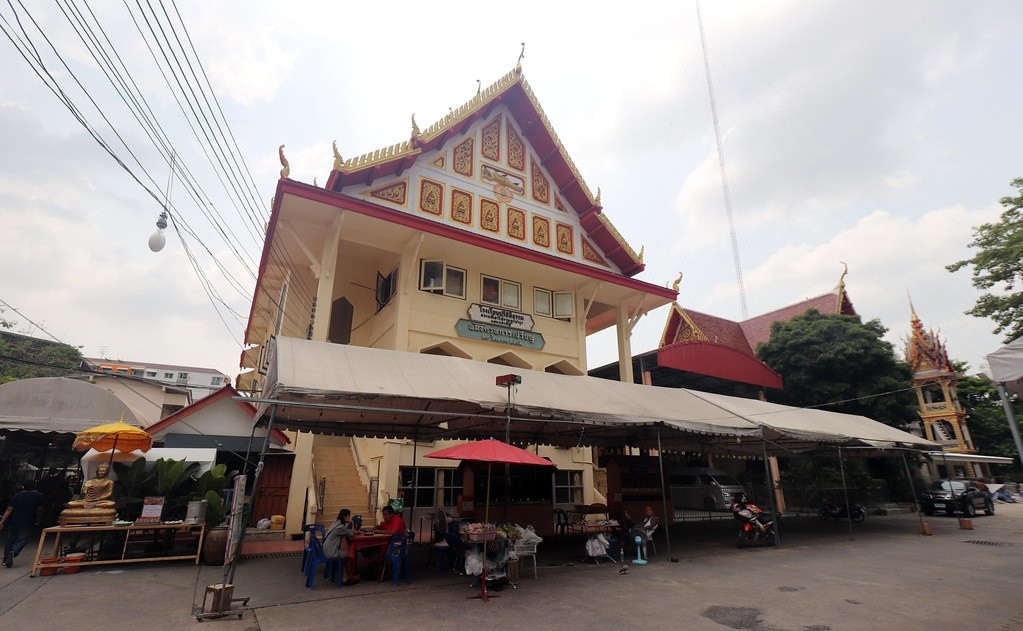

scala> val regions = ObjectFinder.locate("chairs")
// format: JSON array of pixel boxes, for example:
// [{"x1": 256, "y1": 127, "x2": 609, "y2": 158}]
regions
[
  {"x1": 553, "y1": 508, "x2": 575, "y2": 536},
  {"x1": 301, "y1": 523, "x2": 415, "y2": 588},
  {"x1": 626, "y1": 517, "x2": 659, "y2": 559}
]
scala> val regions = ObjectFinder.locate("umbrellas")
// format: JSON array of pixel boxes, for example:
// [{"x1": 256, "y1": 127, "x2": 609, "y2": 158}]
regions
[
  {"x1": 73, "y1": 412, "x2": 153, "y2": 465},
  {"x1": 423, "y1": 437, "x2": 559, "y2": 590}
]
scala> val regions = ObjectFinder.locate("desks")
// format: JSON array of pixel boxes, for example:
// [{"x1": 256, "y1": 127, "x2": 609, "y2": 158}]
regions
[
  {"x1": 420, "y1": 515, "x2": 472, "y2": 544},
  {"x1": 448, "y1": 538, "x2": 516, "y2": 576},
  {"x1": 565, "y1": 509, "x2": 622, "y2": 534},
  {"x1": 31, "y1": 521, "x2": 206, "y2": 577},
  {"x1": 347, "y1": 530, "x2": 392, "y2": 586},
  {"x1": 571, "y1": 523, "x2": 621, "y2": 565},
  {"x1": 516, "y1": 554, "x2": 538, "y2": 580}
]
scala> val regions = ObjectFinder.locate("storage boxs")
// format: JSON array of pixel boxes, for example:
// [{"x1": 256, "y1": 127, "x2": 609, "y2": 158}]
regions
[
  {"x1": 469, "y1": 531, "x2": 495, "y2": 541},
  {"x1": 513, "y1": 544, "x2": 537, "y2": 553},
  {"x1": 456, "y1": 495, "x2": 474, "y2": 517},
  {"x1": 271, "y1": 514, "x2": 285, "y2": 530}
]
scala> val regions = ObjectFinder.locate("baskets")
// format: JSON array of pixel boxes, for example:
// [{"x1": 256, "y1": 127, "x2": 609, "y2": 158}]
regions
[
  {"x1": 512, "y1": 545, "x2": 537, "y2": 554},
  {"x1": 468, "y1": 530, "x2": 496, "y2": 542}
]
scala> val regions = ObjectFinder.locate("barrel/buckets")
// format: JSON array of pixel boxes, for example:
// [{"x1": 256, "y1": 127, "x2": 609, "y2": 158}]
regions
[{"x1": 40, "y1": 553, "x2": 85, "y2": 575}]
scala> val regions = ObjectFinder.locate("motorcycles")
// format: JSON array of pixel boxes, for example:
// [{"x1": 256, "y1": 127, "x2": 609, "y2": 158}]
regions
[
  {"x1": 817, "y1": 499, "x2": 868, "y2": 523},
  {"x1": 731, "y1": 502, "x2": 782, "y2": 550}
]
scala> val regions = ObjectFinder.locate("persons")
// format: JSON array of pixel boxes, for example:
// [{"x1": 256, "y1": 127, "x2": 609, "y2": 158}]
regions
[
  {"x1": 613, "y1": 505, "x2": 659, "y2": 556},
  {"x1": 0, "y1": 480, "x2": 44, "y2": 568},
  {"x1": 68, "y1": 462, "x2": 116, "y2": 508},
  {"x1": 323, "y1": 508, "x2": 359, "y2": 584},
  {"x1": 368, "y1": 505, "x2": 407, "y2": 578}
]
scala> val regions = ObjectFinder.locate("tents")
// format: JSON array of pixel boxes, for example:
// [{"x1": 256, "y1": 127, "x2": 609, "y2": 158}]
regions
[
  {"x1": 224, "y1": 334, "x2": 962, "y2": 606},
  {"x1": 0, "y1": 376, "x2": 142, "y2": 434}
]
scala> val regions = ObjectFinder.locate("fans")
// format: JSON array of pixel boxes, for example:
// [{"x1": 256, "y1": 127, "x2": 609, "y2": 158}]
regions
[{"x1": 631, "y1": 528, "x2": 648, "y2": 564}]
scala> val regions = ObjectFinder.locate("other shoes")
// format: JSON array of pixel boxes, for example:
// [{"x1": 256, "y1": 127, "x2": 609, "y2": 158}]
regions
[
  {"x1": 7, "y1": 551, "x2": 14, "y2": 567},
  {"x1": 1, "y1": 558, "x2": 7, "y2": 567},
  {"x1": 343, "y1": 575, "x2": 360, "y2": 585}
]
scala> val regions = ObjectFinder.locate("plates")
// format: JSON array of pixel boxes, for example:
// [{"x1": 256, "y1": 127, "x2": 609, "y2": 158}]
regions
[{"x1": 112, "y1": 523, "x2": 132, "y2": 526}]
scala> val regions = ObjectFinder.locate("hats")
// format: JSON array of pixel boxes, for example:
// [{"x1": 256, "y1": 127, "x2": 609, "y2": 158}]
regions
[{"x1": 21, "y1": 478, "x2": 34, "y2": 489}]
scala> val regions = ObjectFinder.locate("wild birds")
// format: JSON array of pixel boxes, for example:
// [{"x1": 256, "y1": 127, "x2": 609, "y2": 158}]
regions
[{"x1": 619, "y1": 560, "x2": 630, "y2": 575}]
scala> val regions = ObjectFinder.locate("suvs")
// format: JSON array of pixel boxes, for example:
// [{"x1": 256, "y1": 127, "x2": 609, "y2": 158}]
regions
[{"x1": 919, "y1": 478, "x2": 994, "y2": 518}]
